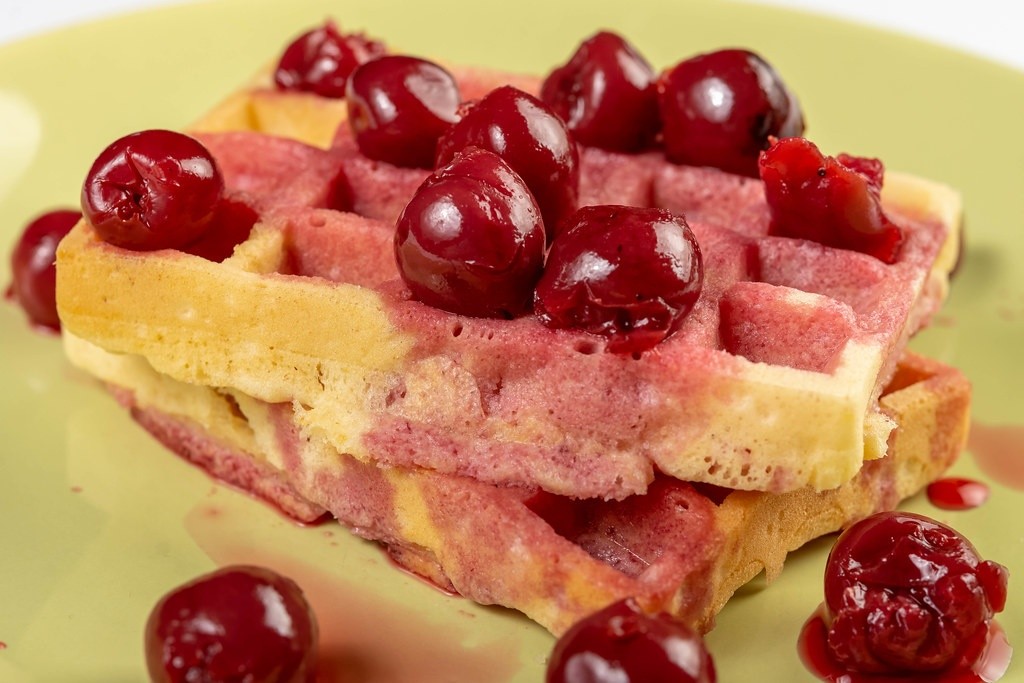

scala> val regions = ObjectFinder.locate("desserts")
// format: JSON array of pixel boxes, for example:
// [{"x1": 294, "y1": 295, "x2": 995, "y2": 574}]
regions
[{"x1": 10, "y1": 18, "x2": 1012, "y2": 683}]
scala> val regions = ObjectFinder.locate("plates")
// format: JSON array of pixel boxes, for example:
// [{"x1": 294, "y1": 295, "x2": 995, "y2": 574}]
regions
[{"x1": 0, "y1": 2, "x2": 1024, "y2": 683}]
